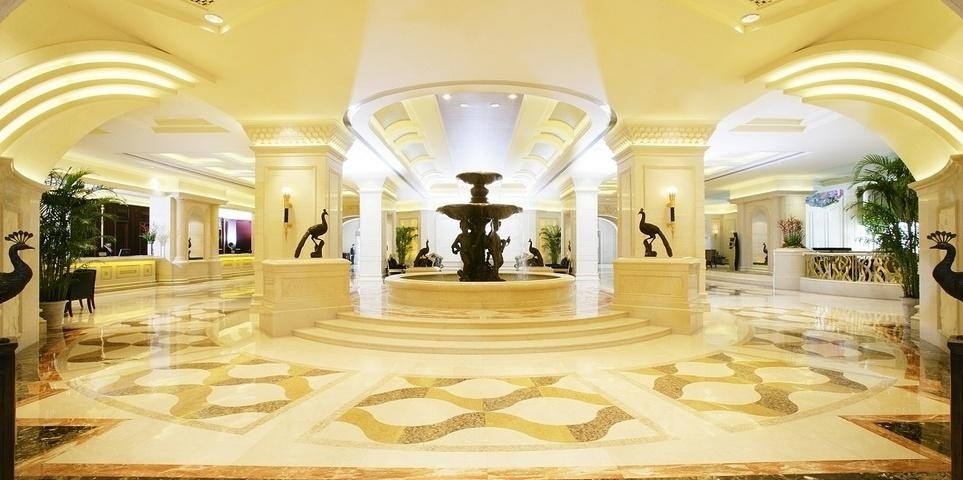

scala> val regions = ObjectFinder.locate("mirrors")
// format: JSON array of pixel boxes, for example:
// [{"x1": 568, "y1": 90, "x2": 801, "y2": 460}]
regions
[
  {"x1": 638, "y1": 208, "x2": 673, "y2": 257},
  {"x1": 528, "y1": 239, "x2": 542, "y2": 259},
  {"x1": 294, "y1": 209, "x2": 328, "y2": 258},
  {"x1": 927, "y1": 230, "x2": 963, "y2": 302},
  {"x1": 0, "y1": 230, "x2": 35, "y2": 304},
  {"x1": 415, "y1": 240, "x2": 429, "y2": 260},
  {"x1": 763, "y1": 242, "x2": 768, "y2": 253}
]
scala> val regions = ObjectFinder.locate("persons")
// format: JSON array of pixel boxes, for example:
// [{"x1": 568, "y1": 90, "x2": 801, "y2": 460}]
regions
[
  {"x1": 103, "y1": 241, "x2": 113, "y2": 256},
  {"x1": 350, "y1": 244, "x2": 355, "y2": 264},
  {"x1": 228, "y1": 242, "x2": 235, "y2": 253}
]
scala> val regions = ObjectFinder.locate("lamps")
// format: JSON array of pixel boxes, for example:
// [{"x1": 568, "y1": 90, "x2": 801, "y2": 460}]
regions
[
  {"x1": 706, "y1": 249, "x2": 718, "y2": 269},
  {"x1": 387, "y1": 256, "x2": 407, "y2": 275},
  {"x1": 67, "y1": 269, "x2": 97, "y2": 317}
]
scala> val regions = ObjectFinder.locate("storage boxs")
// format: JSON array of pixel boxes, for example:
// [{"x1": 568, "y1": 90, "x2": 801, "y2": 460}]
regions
[{"x1": 544, "y1": 257, "x2": 571, "y2": 274}]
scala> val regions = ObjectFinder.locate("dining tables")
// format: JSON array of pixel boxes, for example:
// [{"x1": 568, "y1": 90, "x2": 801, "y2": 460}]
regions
[
  {"x1": 39, "y1": 164, "x2": 128, "y2": 330},
  {"x1": 138, "y1": 231, "x2": 157, "y2": 255},
  {"x1": 844, "y1": 153, "x2": 917, "y2": 327}
]
